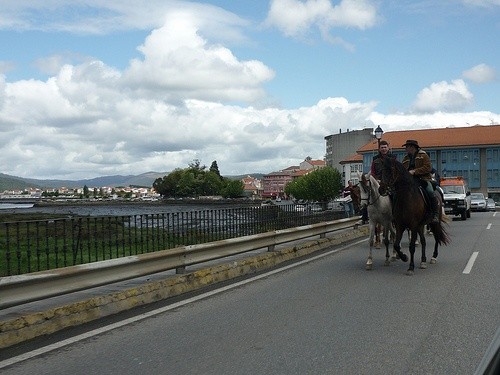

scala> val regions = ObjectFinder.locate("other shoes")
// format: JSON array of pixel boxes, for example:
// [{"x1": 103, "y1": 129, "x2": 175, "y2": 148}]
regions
[{"x1": 431, "y1": 216, "x2": 439, "y2": 224}]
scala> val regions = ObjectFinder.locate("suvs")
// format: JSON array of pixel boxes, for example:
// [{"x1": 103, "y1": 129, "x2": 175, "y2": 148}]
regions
[{"x1": 439, "y1": 176, "x2": 470, "y2": 219}]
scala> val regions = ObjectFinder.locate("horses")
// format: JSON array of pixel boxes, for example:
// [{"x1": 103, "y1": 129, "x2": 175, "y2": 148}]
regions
[{"x1": 357, "y1": 155, "x2": 456, "y2": 275}]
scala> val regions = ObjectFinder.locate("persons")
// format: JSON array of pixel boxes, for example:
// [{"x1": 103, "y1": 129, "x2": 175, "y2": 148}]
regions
[
  {"x1": 425, "y1": 151, "x2": 440, "y2": 191},
  {"x1": 343, "y1": 180, "x2": 354, "y2": 198},
  {"x1": 371, "y1": 141, "x2": 397, "y2": 182},
  {"x1": 401, "y1": 139, "x2": 440, "y2": 223}
]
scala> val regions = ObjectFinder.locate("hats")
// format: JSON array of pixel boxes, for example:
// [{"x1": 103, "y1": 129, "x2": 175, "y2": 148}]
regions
[{"x1": 402, "y1": 140, "x2": 419, "y2": 147}]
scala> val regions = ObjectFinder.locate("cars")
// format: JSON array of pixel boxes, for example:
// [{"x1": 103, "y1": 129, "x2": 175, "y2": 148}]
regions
[
  {"x1": 486, "y1": 198, "x2": 495, "y2": 211},
  {"x1": 470, "y1": 192, "x2": 486, "y2": 211}
]
